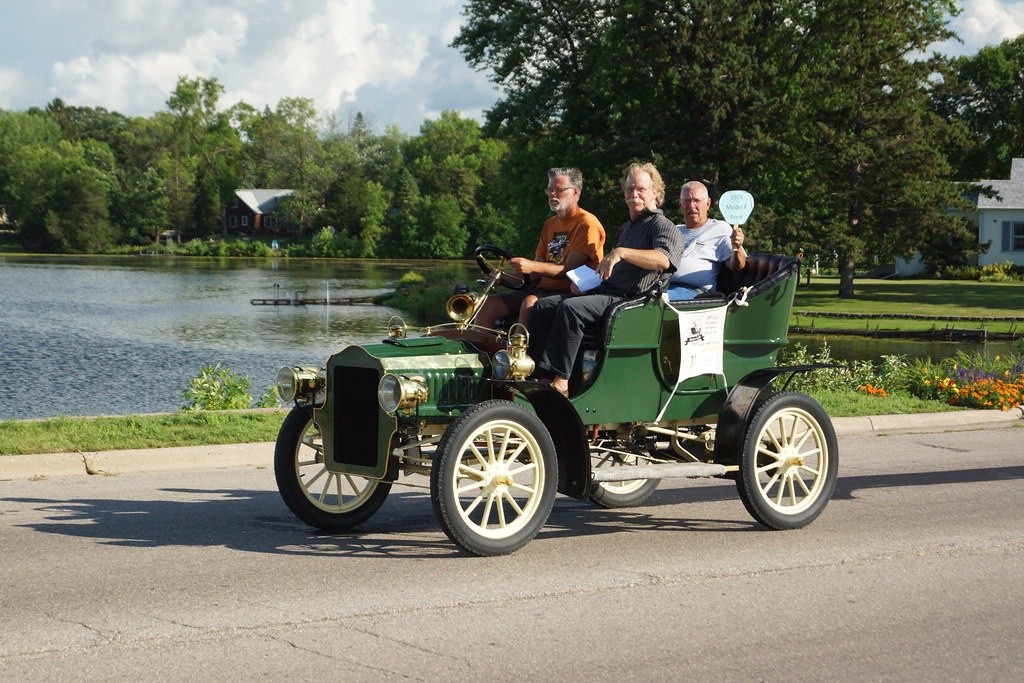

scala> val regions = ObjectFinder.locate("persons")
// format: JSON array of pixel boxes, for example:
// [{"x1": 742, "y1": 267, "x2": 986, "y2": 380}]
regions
[{"x1": 471, "y1": 162, "x2": 747, "y2": 399}]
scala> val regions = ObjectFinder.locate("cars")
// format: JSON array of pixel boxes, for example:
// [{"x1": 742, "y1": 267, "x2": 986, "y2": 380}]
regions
[{"x1": 273, "y1": 245, "x2": 839, "y2": 558}]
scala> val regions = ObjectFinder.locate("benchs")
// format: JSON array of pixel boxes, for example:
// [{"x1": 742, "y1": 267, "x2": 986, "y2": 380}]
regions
[
  {"x1": 717, "y1": 251, "x2": 802, "y2": 303},
  {"x1": 494, "y1": 275, "x2": 670, "y2": 349}
]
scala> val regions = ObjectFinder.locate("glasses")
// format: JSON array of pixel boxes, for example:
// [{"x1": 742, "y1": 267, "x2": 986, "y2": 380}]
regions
[
  {"x1": 681, "y1": 198, "x2": 706, "y2": 206},
  {"x1": 544, "y1": 187, "x2": 576, "y2": 195},
  {"x1": 623, "y1": 186, "x2": 652, "y2": 194}
]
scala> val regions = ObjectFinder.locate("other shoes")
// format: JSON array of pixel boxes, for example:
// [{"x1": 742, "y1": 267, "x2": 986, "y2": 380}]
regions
[{"x1": 546, "y1": 381, "x2": 569, "y2": 399}]
